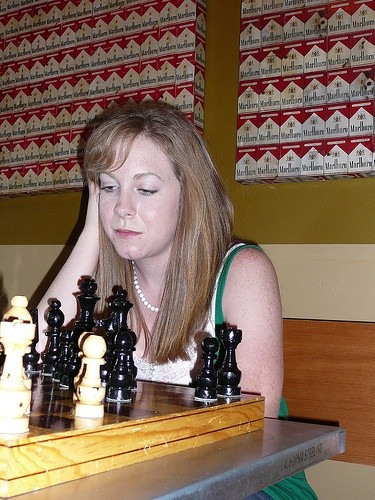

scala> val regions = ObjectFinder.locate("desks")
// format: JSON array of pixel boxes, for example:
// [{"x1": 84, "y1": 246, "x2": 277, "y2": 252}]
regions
[{"x1": 2, "y1": 418, "x2": 344, "y2": 500}]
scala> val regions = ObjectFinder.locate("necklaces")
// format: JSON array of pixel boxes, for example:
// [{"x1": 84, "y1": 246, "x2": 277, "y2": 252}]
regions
[{"x1": 132, "y1": 261, "x2": 160, "y2": 312}]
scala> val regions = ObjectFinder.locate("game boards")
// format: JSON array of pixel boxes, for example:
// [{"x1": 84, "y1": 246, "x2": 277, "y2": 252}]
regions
[{"x1": 0, "y1": 376, "x2": 265, "y2": 498}]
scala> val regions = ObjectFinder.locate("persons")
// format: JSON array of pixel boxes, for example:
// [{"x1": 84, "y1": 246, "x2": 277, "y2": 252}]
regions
[{"x1": 35, "y1": 100, "x2": 320, "y2": 500}]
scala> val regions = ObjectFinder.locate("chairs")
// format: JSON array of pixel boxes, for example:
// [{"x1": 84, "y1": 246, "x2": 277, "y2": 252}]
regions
[{"x1": 280, "y1": 318, "x2": 375, "y2": 465}]
